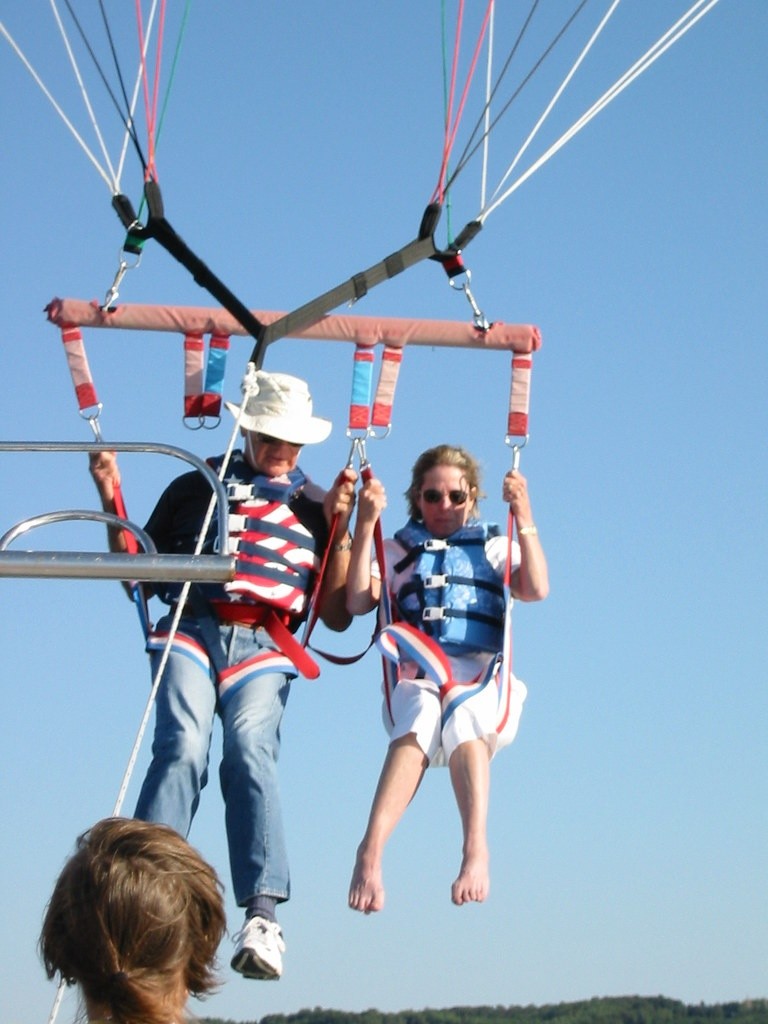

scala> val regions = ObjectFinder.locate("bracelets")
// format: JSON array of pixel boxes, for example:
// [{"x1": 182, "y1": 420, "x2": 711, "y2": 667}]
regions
[{"x1": 517, "y1": 526, "x2": 538, "y2": 538}]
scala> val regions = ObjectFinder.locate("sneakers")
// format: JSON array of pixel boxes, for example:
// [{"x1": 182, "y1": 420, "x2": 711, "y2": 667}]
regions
[{"x1": 230, "y1": 918, "x2": 285, "y2": 981}]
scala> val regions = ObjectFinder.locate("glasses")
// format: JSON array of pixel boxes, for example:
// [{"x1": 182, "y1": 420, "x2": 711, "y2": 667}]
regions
[
  {"x1": 419, "y1": 489, "x2": 471, "y2": 505},
  {"x1": 256, "y1": 433, "x2": 305, "y2": 447}
]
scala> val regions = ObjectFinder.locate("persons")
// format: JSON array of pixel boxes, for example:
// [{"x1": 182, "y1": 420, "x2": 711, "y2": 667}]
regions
[
  {"x1": 33, "y1": 812, "x2": 231, "y2": 1024},
  {"x1": 87, "y1": 369, "x2": 359, "y2": 982},
  {"x1": 349, "y1": 443, "x2": 551, "y2": 916}
]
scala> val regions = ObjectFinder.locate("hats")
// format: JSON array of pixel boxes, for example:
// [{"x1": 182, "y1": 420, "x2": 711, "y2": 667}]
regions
[{"x1": 224, "y1": 370, "x2": 333, "y2": 445}]
